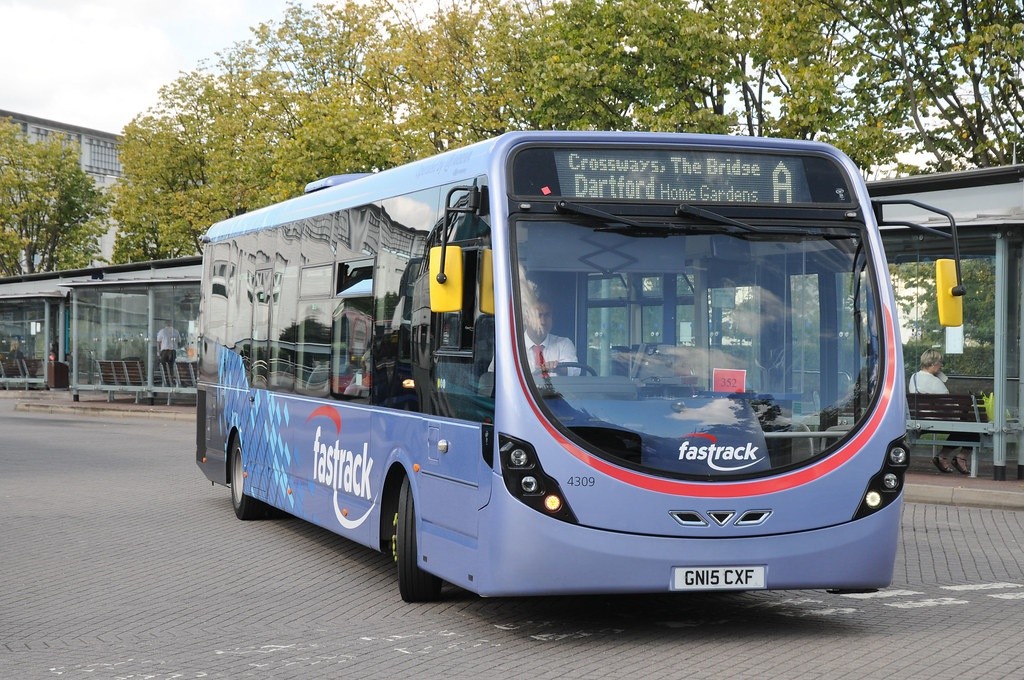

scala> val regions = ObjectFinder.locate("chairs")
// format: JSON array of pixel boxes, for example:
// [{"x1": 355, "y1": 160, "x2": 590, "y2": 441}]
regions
[{"x1": 473, "y1": 313, "x2": 494, "y2": 385}]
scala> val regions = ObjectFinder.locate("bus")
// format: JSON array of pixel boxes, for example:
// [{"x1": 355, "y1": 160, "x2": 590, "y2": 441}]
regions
[{"x1": 195, "y1": 132, "x2": 969, "y2": 603}]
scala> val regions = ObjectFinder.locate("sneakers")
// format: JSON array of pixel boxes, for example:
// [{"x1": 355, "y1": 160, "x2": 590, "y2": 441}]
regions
[
  {"x1": 933, "y1": 454, "x2": 952, "y2": 473},
  {"x1": 951, "y1": 456, "x2": 971, "y2": 474}
]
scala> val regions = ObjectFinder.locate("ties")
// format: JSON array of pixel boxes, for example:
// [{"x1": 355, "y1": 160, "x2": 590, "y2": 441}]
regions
[{"x1": 533, "y1": 344, "x2": 549, "y2": 379}]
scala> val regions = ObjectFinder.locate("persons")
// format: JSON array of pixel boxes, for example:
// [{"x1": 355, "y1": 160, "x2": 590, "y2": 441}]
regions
[
  {"x1": 157, "y1": 320, "x2": 181, "y2": 386},
  {"x1": 53, "y1": 336, "x2": 67, "y2": 361},
  {"x1": 908, "y1": 348, "x2": 979, "y2": 474},
  {"x1": 9, "y1": 341, "x2": 24, "y2": 359},
  {"x1": 486, "y1": 300, "x2": 582, "y2": 377},
  {"x1": 344, "y1": 348, "x2": 370, "y2": 397}
]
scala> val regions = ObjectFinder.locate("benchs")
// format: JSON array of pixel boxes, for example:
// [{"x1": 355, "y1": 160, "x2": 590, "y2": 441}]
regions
[
  {"x1": 162, "y1": 360, "x2": 199, "y2": 406},
  {"x1": 844, "y1": 389, "x2": 991, "y2": 478},
  {"x1": 93, "y1": 359, "x2": 161, "y2": 405},
  {"x1": 0, "y1": 357, "x2": 45, "y2": 391}
]
